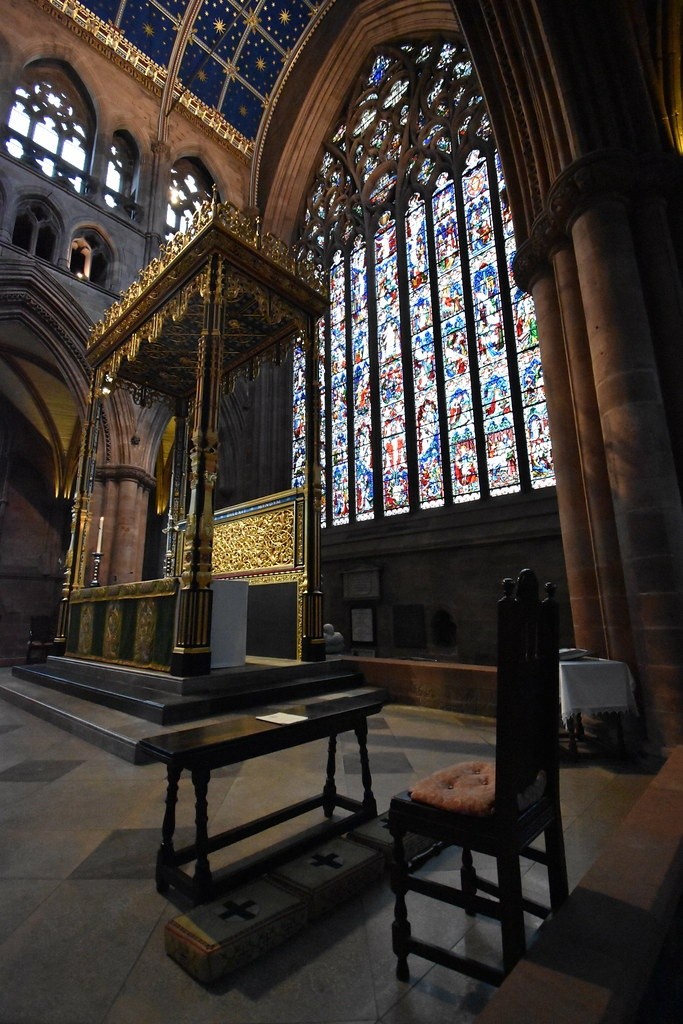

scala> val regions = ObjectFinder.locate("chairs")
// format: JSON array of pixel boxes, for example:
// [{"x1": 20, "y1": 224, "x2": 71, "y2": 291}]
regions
[
  {"x1": 25, "y1": 615, "x2": 53, "y2": 665},
  {"x1": 388, "y1": 568, "x2": 562, "y2": 989}
]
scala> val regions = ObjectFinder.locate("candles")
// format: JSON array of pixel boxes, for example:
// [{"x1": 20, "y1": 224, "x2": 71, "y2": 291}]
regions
[{"x1": 97, "y1": 517, "x2": 104, "y2": 553}]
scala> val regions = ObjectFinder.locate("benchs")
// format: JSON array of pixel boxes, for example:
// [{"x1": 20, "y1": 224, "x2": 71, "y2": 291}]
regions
[{"x1": 139, "y1": 695, "x2": 383, "y2": 908}]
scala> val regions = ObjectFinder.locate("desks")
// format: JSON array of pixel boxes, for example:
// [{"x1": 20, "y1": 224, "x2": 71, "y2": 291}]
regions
[{"x1": 559, "y1": 656, "x2": 638, "y2": 762}]
